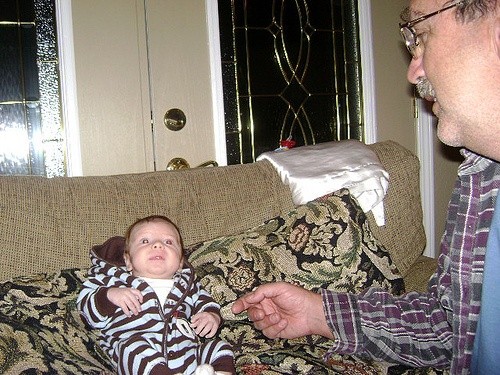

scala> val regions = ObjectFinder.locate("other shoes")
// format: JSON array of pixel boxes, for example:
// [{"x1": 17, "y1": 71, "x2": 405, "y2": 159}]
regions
[{"x1": 194, "y1": 364, "x2": 214, "y2": 375}]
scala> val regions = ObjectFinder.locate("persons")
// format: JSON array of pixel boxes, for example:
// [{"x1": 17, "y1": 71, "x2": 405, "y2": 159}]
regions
[
  {"x1": 231, "y1": 0, "x2": 500, "y2": 375},
  {"x1": 75, "y1": 214, "x2": 235, "y2": 375}
]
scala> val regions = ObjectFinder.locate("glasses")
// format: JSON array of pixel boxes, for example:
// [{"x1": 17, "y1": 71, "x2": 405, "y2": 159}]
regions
[{"x1": 398, "y1": 0, "x2": 467, "y2": 51}]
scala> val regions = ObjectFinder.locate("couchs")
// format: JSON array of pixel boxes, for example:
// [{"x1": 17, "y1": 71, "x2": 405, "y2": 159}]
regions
[{"x1": 0, "y1": 140, "x2": 449, "y2": 375}]
